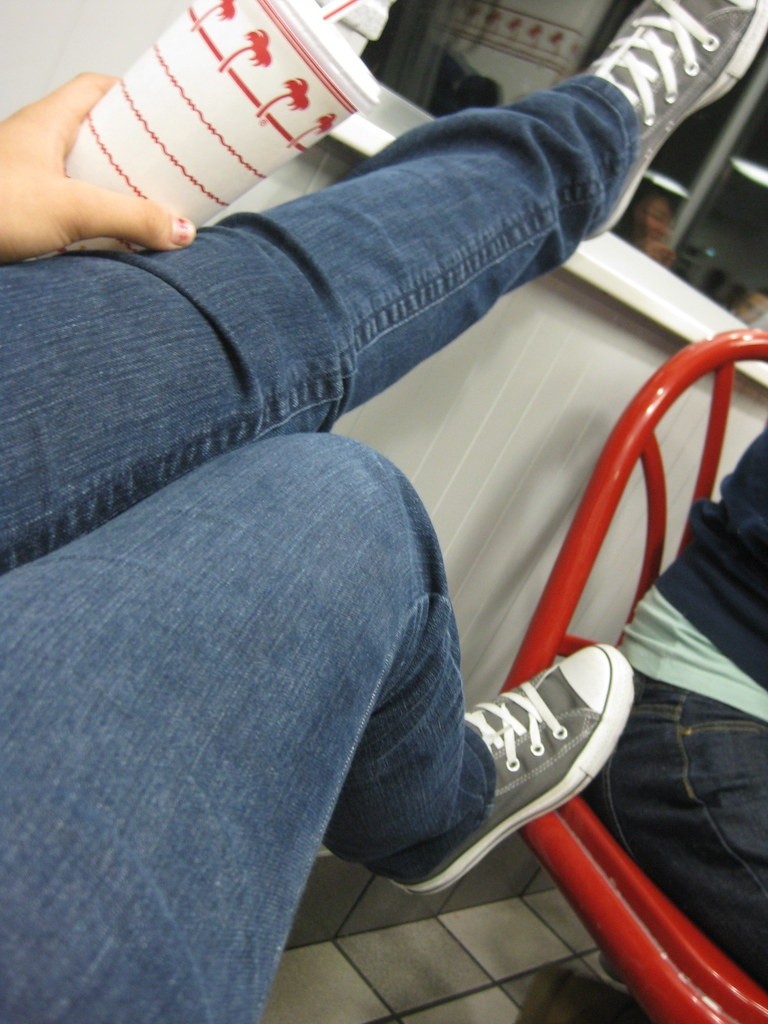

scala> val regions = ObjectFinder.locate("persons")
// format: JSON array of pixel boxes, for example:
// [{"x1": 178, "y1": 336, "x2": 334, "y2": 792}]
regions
[
  {"x1": 580, "y1": 419, "x2": 768, "y2": 993},
  {"x1": 0, "y1": 0, "x2": 768, "y2": 1024}
]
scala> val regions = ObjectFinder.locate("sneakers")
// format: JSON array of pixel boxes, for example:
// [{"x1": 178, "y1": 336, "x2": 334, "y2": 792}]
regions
[
  {"x1": 383, "y1": 644, "x2": 634, "y2": 896},
  {"x1": 582, "y1": 0, "x2": 768, "y2": 241}
]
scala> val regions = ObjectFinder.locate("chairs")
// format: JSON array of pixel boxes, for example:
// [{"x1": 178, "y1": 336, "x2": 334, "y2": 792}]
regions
[{"x1": 492, "y1": 326, "x2": 768, "y2": 1024}]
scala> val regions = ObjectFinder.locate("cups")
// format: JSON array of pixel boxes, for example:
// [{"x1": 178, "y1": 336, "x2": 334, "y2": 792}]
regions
[{"x1": 36, "y1": 0, "x2": 379, "y2": 262}]
